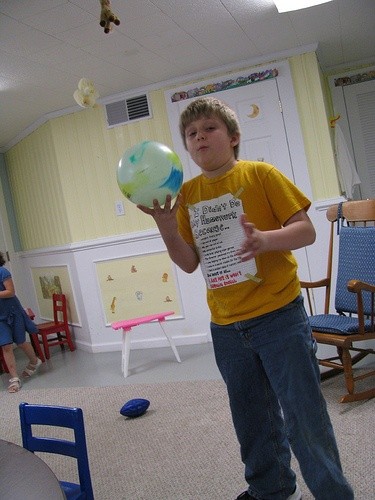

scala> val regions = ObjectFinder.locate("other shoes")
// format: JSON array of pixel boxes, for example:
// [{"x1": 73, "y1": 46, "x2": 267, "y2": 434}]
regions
[{"x1": 234, "y1": 482, "x2": 302, "y2": 500}]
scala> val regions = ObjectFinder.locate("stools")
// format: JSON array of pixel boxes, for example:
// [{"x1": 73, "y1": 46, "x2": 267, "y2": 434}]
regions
[{"x1": 111, "y1": 310, "x2": 182, "y2": 377}]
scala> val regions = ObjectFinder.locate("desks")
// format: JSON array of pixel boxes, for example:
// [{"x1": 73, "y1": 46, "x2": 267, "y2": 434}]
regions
[{"x1": 0, "y1": 307, "x2": 46, "y2": 375}]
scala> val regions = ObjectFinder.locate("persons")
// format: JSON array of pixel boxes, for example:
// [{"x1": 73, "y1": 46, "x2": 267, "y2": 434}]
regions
[
  {"x1": 0, "y1": 250, "x2": 42, "y2": 393},
  {"x1": 137, "y1": 97, "x2": 355, "y2": 500}
]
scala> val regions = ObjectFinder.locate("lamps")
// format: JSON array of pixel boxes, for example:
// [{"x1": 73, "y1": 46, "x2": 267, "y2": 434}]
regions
[{"x1": 272, "y1": 0, "x2": 334, "y2": 14}]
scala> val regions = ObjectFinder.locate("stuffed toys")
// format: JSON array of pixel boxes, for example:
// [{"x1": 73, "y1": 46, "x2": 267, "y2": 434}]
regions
[{"x1": 99, "y1": 0, "x2": 120, "y2": 34}]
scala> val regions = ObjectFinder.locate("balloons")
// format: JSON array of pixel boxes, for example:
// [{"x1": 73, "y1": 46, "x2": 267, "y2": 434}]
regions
[{"x1": 117, "y1": 139, "x2": 184, "y2": 208}]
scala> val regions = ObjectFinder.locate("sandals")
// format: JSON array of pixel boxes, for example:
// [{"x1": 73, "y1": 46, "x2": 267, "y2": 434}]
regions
[
  {"x1": 22, "y1": 357, "x2": 42, "y2": 377},
  {"x1": 8, "y1": 377, "x2": 21, "y2": 392}
]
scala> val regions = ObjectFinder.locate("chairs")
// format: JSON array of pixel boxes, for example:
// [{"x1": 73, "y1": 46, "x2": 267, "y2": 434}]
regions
[
  {"x1": 37, "y1": 292, "x2": 76, "y2": 360},
  {"x1": 299, "y1": 197, "x2": 375, "y2": 404},
  {"x1": 18, "y1": 401, "x2": 94, "y2": 500}
]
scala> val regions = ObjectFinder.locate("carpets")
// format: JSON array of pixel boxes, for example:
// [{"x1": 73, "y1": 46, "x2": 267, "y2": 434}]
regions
[{"x1": 0, "y1": 367, "x2": 375, "y2": 500}]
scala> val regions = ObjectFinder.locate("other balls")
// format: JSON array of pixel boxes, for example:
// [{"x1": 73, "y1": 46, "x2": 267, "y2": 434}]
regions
[{"x1": 116, "y1": 140, "x2": 184, "y2": 209}]
